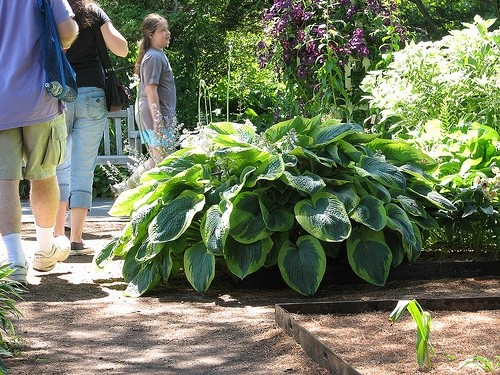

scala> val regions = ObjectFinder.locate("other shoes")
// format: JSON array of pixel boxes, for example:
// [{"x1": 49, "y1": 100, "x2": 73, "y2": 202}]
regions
[
  {"x1": 114, "y1": 180, "x2": 127, "y2": 195},
  {"x1": 70, "y1": 241, "x2": 95, "y2": 255}
]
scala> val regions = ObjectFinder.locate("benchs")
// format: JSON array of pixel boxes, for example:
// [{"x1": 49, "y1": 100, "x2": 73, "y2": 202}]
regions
[{"x1": 22, "y1": 106, "x2": 143, "y2": 168}]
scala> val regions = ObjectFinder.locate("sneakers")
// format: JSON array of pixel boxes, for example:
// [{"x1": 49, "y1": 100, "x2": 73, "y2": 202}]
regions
[
  {"x1": 7, "y1": 260, "x2": 30, "y2": 286},
  {"x1": 33, "y1": 235, "x2": 71, "y2": 272}
]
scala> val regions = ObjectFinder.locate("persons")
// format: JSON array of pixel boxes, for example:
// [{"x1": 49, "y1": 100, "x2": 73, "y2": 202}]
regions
[
  {"x1": 51, "y1": 0, "x2": 129, "y2": 256},
  {"x1": 0, "y1": 0, "x2": 79, "y2": 285},
  {"x1": 112, "y1": 15, "x2": 177, "y2": 199}
]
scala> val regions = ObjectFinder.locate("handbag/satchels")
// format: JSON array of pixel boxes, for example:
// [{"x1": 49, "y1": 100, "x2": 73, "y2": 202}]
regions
[
  {"x1": 91, "y1": 19, "x2": 129, "y2": 112},
  {"x1": 37, "y1": 0, "x2": 78, "y2": 103}
]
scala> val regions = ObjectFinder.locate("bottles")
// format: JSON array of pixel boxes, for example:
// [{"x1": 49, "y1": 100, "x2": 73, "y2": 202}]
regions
[{"x1": 45, "y1": 81, "x2": 77, "y2": 103}]
